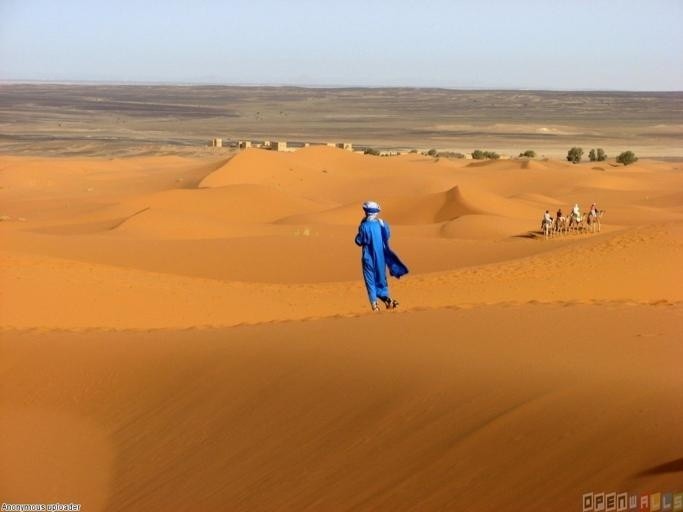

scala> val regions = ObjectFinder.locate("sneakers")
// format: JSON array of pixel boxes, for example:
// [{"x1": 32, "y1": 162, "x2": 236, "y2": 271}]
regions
[
  {"x1": 371, "y1": 304, "x2": 380, "y2": 312},
  {"x1": 383, "y1": 300, "x2": 399, "y2": 309}
]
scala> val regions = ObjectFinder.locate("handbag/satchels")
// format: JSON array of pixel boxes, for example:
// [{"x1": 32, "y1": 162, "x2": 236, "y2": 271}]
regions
[{"x1": 383, "y1": 246, "x2": 409, "y2": 280}]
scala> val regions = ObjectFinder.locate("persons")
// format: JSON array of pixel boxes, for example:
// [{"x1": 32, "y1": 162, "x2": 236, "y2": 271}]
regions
[
  {"x1": 555, "y1": 208, "x2": 562, "y2": 224},
  {"x1": 355, "y1": 200, "x2": 409, "y2": 311},
  {"x1": 573, "y1": 204, "x2": 580, "y2": 219},
  {"x1": 590, "y1": 202, "x2": 599, "y2": 218},
  {"x1": 541, "y1": 210, "x2": 553, "y2": 229}
]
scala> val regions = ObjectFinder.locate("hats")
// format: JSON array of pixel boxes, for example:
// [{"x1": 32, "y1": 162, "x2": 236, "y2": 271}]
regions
[{"x1": 362, "y1": 200, "x2": 381, "y2": 213}]
